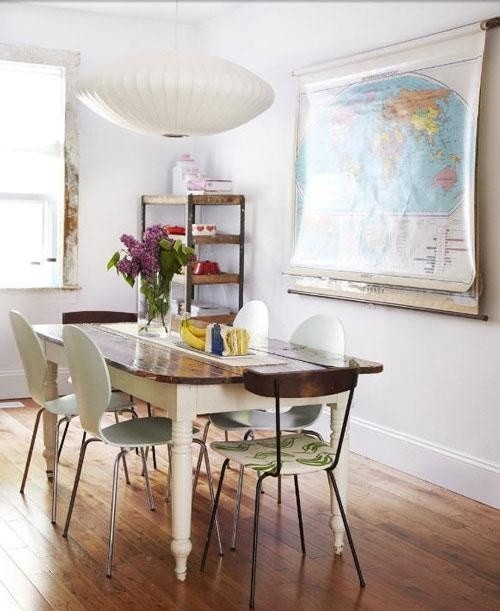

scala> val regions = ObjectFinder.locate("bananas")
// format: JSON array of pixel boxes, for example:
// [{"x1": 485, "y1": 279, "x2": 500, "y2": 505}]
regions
[{"x1": 180, "y1": 317, "x2": 206, "y2": 350}]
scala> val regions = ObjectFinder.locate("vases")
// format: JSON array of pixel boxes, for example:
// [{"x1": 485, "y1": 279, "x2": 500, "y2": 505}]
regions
[{"x1": 136, "y1": 274, "x2": 172, "y2": 335}]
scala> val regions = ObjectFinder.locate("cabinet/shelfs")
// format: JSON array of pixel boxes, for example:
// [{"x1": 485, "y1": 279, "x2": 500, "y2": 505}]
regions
[{"x1": 136, "y1": 194, "x2": 248, "y2": 321}]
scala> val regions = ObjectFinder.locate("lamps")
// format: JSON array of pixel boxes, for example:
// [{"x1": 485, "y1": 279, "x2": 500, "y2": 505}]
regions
[{"x1": 73, "y1": 0, "x2": 278, "y2": 143}]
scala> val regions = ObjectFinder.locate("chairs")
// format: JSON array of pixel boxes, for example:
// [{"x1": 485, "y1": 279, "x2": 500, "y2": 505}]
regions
[
  {"x1": 193, "y1": 315, "x2": 346, "y2": 551},
  {"x1": 201, "y1": 360, "x2": 367, "y2": 611},
  {"x1": 8, "y1": 310, "x2": 155, "y2": 525},
  {"x1": 61, "y1": 325, "x2": 223, "y2": 579},
  {"x1": 58, "y1": 310, "x2": 157, "y2": 470},
  {"x1": 141, "y1": 300, "x2": 268, "y2": 494}
]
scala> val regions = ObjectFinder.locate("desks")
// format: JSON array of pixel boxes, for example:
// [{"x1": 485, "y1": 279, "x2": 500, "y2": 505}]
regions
[{"x1": 36, "y1": 319, "x2": 385, "y2": 585}]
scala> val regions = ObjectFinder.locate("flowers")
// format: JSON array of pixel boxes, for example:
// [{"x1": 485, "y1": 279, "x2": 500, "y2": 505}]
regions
[{"x1": 105, "y1": 222, "x2": 196, "y2": 334}]
